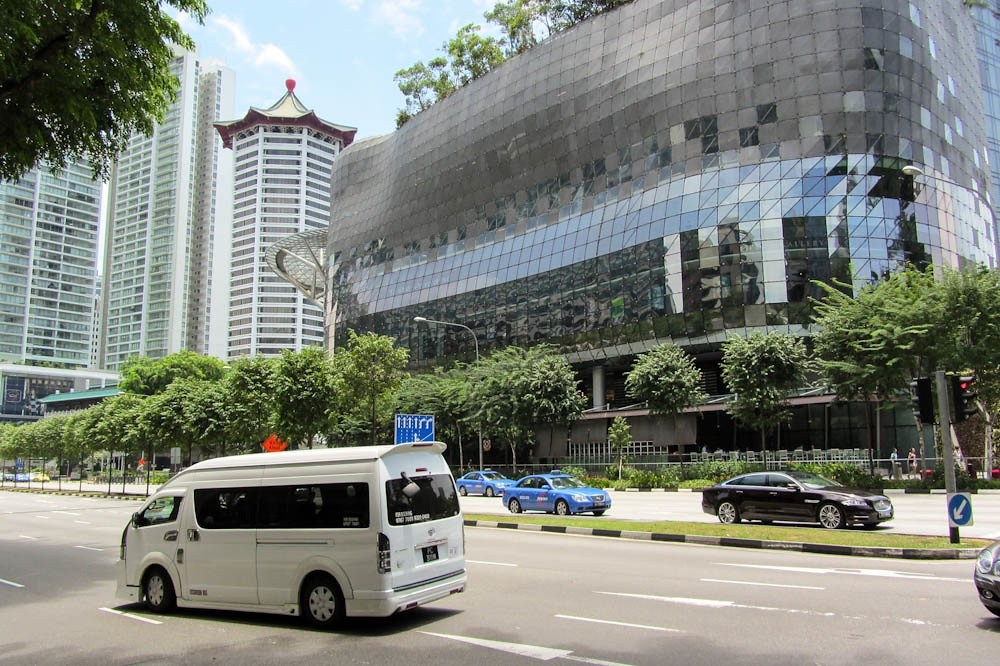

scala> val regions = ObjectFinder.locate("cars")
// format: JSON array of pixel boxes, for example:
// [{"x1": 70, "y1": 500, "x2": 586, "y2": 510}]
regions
[
  {"x1": 455, "y1": 470, "x2": 517, "y2": 496},
  {"x1": 502, "y1": 470, "x2": 612, "y2": 516},
  {"x1": 974, "y1": 540, "x2": 1000, "y2": 616},
  {"x1": 701, "y1": 468, "x2": 896, "y2": 530},
  {"x1": 0, "y1": 471, "x2": 50, "y2": 483}
]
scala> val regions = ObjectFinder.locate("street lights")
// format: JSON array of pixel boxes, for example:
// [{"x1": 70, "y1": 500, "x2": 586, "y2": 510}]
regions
[
  {"x1": 413, "y1": 315, "x2": 483, "y2": 470},
  {"x1": 901, "y1": 164, "x2": 1000, "y2": 279}
]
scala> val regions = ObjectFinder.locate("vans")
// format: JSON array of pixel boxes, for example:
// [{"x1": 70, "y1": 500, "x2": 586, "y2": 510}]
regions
[{"x1": 117, "y1": 439, "x2": 469, "y2": 631}]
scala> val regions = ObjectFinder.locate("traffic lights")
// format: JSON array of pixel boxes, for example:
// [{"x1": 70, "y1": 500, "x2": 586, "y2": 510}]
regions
[
  {"x1": 950, "y1": 375, "x2": 980, "y2": 423},
  {"x1": 910, "y1": 377, "x2": 935, "y2": 424}
]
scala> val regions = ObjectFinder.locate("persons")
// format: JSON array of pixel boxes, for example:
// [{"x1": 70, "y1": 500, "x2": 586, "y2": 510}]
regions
[
  {"x1": 889, "y1": 448, "x2": 897, "y2": 479},
  {"x1": 954, "y1": 446, "x2": 962, "y2": 463},
  {"x1": 908, "y1": 447, "x2": 917, "y2": 479},
  {"x1": 539, "y1": 479, "x2": 546, "y2": 488}
]
